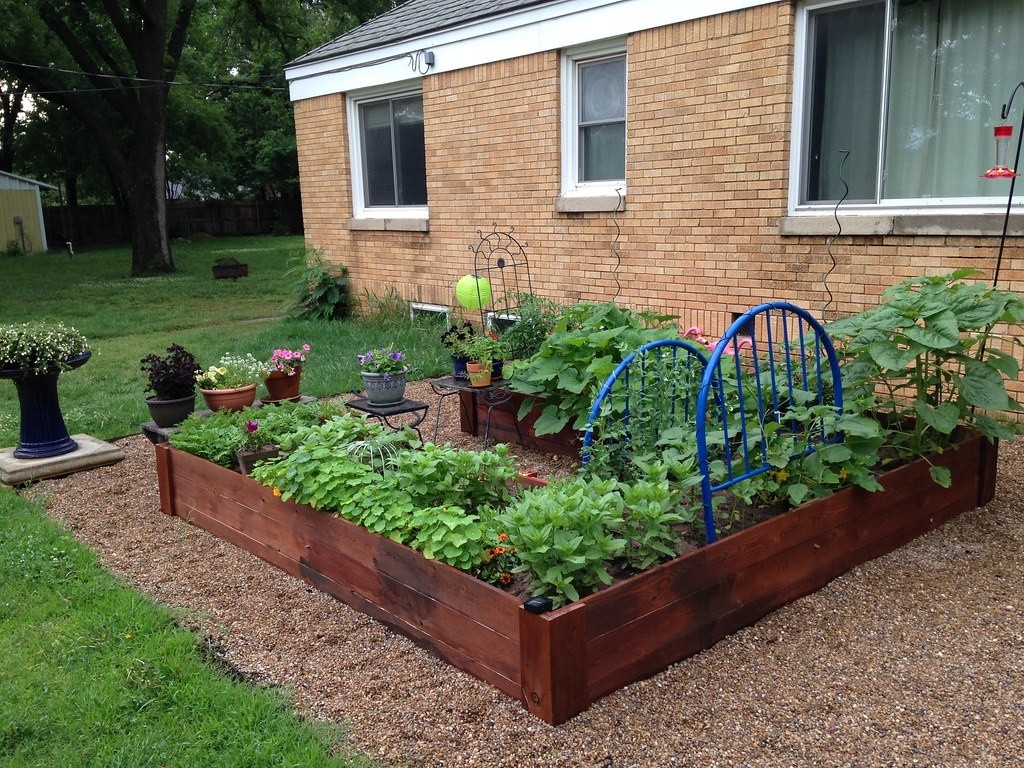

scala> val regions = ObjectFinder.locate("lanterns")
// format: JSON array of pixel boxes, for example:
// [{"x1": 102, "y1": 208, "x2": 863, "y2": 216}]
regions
[{"x1": 456, "y1": 275, "x2": 492, "y2": 309}]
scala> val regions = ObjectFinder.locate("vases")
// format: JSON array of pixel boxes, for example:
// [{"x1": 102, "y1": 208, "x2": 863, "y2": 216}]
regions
[
  {"x1": 0, "y1": 351, "x2": 92, "y2": 459},
  {"x1": 199, "y1": 382, "x2": 259, "y2": 415},
  {"x1": 235, "y1": 441, "x2": 278, "y2": 476},
  {"x1": 264, "y1": 366, "x2": 302, "y2": 400},
  {"x1": 360, "y1": 366, "x2": 408, "y2": 407}
]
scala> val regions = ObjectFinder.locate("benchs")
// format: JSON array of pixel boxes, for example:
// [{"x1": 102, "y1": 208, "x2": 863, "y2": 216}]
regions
[{"x1": 140, "y1": 396, "x2": 317, "y2": 445}]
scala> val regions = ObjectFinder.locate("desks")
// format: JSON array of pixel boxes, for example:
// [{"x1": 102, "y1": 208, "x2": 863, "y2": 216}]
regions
[
  {"x1": 344, "y1": 397, "x2": 430, "y2": 444},
  {"x1": 430, "y1": 377, "x2": 522, "y2": 450}
]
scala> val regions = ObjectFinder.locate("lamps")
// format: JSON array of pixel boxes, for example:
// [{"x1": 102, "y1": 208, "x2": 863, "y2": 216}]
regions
[{"x1": 967, "y1": 82, "x2": 1024, "y2": 503}]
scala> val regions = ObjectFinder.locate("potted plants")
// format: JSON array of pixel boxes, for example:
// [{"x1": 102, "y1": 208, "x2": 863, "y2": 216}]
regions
[
  {"x1": 440, "y1": 320, "x2": 473, "y2": 379},
  {"x1": 140, "y1": 343, "x2": 201, "y2": 429},
  {"x1": 462, "y1": 338, "x2": 493, "y2": 388},
  {"x1": 491, "y1": 341, "x2": 512, "y2": 380}
]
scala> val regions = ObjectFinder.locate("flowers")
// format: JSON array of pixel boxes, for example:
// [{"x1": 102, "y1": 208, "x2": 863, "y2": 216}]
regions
[
  {"x1": 358, "y1": 343, "x2": 403, "y2": 381},
  {"x1": 0, "y1": 321, "x2": 101, "y2": 375},
  {"x1": 193, "y1": 352, "x2": 270, "y2": 391},
  {"x1": 237, "y1": 418, "x2": 274, "y2": 452},
  {"x1": 270, "y1": 343, "x2": 310, "y2": 376},
  {"x1": 486, "y1": 534, "x2": 515, "y2": 586}
]
